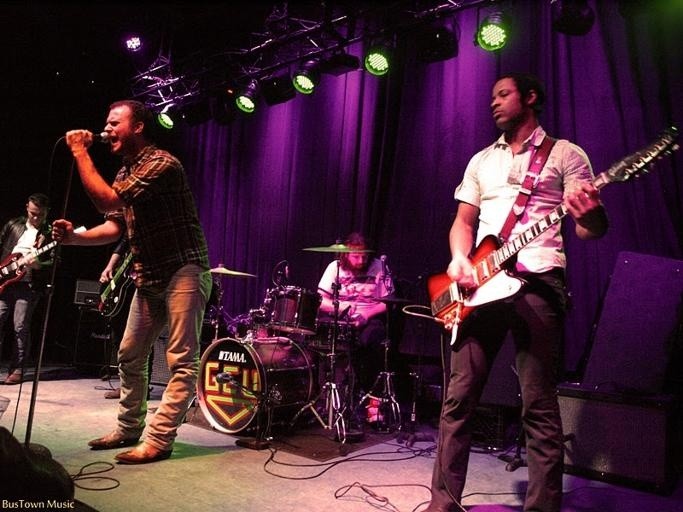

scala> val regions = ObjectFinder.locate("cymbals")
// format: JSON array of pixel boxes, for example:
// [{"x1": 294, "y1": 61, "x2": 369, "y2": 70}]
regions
[
  {"x1": 373, "y1": 293, "x2": 417, "y2": 305},
  {"x1": 301, "y1": 244, "x2": 379, "y2": 258},
  {"x1": 207, "y1": 265, "x2": 262, "y2": 279}
]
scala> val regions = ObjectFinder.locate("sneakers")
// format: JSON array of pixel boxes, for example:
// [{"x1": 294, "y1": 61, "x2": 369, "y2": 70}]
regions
[{"x1": 365, "y1": 400, "x2": 384, "y2": 423}]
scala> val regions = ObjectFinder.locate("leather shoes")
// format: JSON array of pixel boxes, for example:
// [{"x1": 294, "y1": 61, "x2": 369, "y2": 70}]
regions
[
  {"x1": 5, "y1": 374, "x2": 22, "y2": 385},
  {"x1": 88, "y1": 431, "x2": 139, "y2": 447},
  {"x1": 423, "y1": 507, "x2": 456, "y2": 512},
  {"x1": 114, "y1": 442, "x2": 173, "y2": 464},
  {"x1": 105, "y1": 388, "x2": 120, "y2": 399}
]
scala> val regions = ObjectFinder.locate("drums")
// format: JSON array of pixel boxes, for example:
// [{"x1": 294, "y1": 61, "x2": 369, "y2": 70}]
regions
[
  {"x1": 263, "y1": 284, "x2": 323, "y2": 335},
  {"x1": 194, "y1": 331, "x2": 315, "y2": 439},
  {"x1": 311, "y1": 315, "x2": 360, "y2": 353}
]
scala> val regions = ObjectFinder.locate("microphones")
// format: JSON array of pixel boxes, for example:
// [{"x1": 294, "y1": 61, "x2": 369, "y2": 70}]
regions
[
  {"x1": 380, "y1": 255, "x2": 386, "y2": 285},
  {"x1": 90, "y1": 132, "x2": 108, "y2": 139}
]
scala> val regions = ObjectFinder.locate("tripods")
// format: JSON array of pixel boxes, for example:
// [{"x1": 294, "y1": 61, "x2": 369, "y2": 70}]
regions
[
  {"x1": 286, "y1": 257, "x2": 364, "y2": 458},
  {"x1": 352, "y1": 302, "x2": 404, "y2": 443},
  {"x1": 178, "y1": 287, "x2": 222, "y2": 431}
]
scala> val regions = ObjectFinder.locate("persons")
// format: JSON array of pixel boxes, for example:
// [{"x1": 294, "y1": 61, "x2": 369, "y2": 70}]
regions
[
  {"x1": 50, "y1": 101, "x2": 212, "y2": 464},
  {"x1": 319, "y1": 234, "x2": 396, "y2": 421},
  {"x1": 0, "y1": 196, "x2": 57, "y2": 385},
  {"x1": 97, "y1": 227, "x2": 155, "y2": 399},
  {"x1": 423, "y1": 75, "x2": 609, "y2": 512}
]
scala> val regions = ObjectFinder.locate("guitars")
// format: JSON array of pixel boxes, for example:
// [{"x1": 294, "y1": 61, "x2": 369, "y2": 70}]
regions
[
  {"x1": 97, "y1": 248, "x2": 134, "y2": 318},
  {"x1": 422, "y1": 127, "x2": 683, "y2": 350},
  {"x1": 0, "y1": 241, "x2": 60, "y2": 290}
]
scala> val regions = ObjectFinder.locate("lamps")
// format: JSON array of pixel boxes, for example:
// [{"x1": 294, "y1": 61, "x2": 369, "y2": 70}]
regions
[
  {"x1": 156, "y1": 103, "x2": 185, "y2": 133},
  {"x1": 235, "y1": 78, "x2": 260, "y2": 116},
  {"x1": 294, "y1": 57, "x2": 322, "y2": 97},
  {"x1": 364, "y1": 31, "x2": 407, "y2": 75},
  {"x1": 553, "y1": 0, "x2": 594, "y2": 37},
  {"x1": 476, "y1": 10, "x2": 513, "y2": 53}
]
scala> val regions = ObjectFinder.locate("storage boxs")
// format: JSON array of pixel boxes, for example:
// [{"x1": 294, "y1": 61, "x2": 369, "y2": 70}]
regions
[{"x1": 550, "y1": 377, "x2": 683, "y2": 492}]
